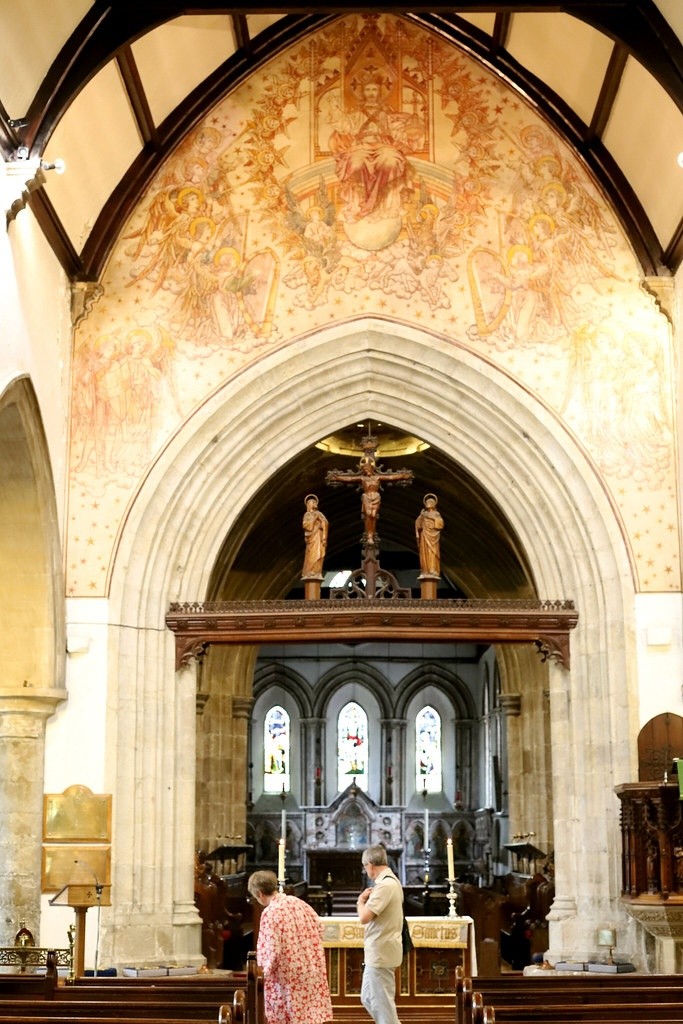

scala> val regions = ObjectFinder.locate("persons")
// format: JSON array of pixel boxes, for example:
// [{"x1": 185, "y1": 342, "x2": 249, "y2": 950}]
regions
[
  {"x1": 415, "y1": 493, "x2": 445, "y2": 577},
  {"x1": 246, "y1": 870, "x2": 333, "y2": 1024},
  {"x1": 356, "y1": 845, "x2": 404, "y2": 1024},
  {"x1": 302, "y1": 494, "x2": 329, "y2": 576},
  {"x1": 330, "y1": 457, "x2": 413, "y2": 546}
]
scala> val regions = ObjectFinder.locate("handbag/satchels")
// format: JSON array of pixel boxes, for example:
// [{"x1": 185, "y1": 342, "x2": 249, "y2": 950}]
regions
[{"x1": 401, "y1": 918, "x2": 415, "y2": 954}]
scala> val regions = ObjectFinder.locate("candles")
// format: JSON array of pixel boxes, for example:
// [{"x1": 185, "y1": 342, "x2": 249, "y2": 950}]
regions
[
  {"x1": 282, "y1": 809, "x2": 286, "y2": 840},
  {"x1": 423, "y1": 808, "x2": 429, "y2": 848},
  {"x1": 278, "y1": 839, "x2": 286, "y2": 880},
  {"x1": 447, "y1": 838, "x2": 455, "y2": 878}
]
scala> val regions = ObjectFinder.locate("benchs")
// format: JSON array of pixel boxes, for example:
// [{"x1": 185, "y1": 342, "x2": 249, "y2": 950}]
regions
[
  {"x1": 453, "y1": 873, "x2": 683, "y2": 1024},
  {"x1": 0, "y1": 874, "x2": 264, "y2": 1024}
]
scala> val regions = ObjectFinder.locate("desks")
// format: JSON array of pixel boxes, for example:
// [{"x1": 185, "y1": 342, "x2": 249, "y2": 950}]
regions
[{"x1": 318, "y1": 915, "x2": 478, "y2": 1012}]
